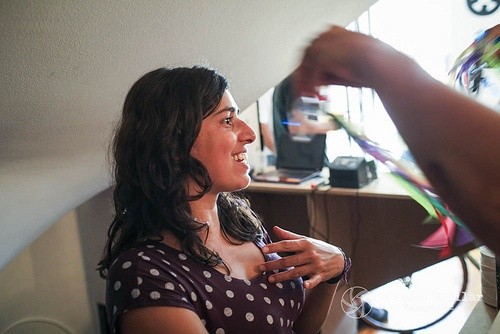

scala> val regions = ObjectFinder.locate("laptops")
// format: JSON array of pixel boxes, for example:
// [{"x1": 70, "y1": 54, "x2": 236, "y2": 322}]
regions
[{"x1": 254, "y1": 134, "x2": 326, "y2": 184}]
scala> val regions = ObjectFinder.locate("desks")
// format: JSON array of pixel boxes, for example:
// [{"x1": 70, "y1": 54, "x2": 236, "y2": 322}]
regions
[
  {"x1": 331, "y1": 246, "x2": 500, "y2": 334},
  {"x1": 244, "y1": 168, "x2": 469, "y2": 295}
]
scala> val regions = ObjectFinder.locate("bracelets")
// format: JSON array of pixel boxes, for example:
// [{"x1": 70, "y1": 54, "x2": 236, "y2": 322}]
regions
[{"x1": 326, "y1": 246, "x2": 351, "y2": 282}]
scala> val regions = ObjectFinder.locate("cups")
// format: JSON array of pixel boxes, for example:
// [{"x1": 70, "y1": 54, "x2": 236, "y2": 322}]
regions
[{"x1": 480, "y1": 246, "x2": 497, "y2": 307}]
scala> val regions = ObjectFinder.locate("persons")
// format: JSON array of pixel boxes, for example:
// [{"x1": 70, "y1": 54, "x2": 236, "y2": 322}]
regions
[
  {"x1": 98, "y1": 66, "x2": 352, "y2": 334},
  {"x1": 292, "y1": 26, "x2": 500, "y2": 258},
  {"x1": 260, "y1": 64, "x2": 342, "y2": 169}
]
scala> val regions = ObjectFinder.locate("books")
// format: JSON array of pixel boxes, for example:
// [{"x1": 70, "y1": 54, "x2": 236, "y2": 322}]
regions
[{"x1": 254, "y1": 168, "x2": 319, "y2": 184}]
